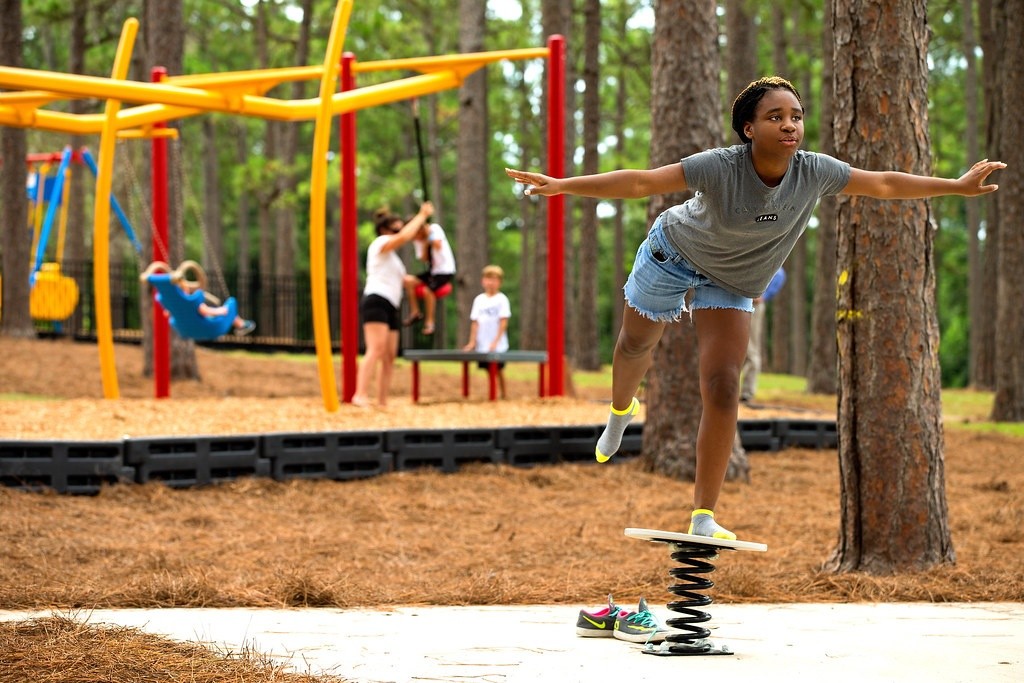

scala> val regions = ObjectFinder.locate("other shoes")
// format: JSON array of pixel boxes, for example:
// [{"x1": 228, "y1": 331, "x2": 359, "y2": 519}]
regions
[
  {"x1": 421, "y1": 323, "x2": 435, "y2": 336},
  {"x1": 402, "y1": 311, "x2": 423, "y2": 327},
  {"x1": 235, "y1": 320, "x2": 256, "y2": 335}
]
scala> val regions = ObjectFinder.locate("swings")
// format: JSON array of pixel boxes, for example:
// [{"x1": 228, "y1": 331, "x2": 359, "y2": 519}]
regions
[{"x1": 118, "y1": 139, "x2": 239, "y2": 341}]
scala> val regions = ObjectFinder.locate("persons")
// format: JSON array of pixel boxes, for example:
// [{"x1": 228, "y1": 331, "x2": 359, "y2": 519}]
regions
[
  {"x1": 505, "y1": 77, "x2": 1007, "y2": 541},
  {"x1": 162, "y1": 272, "x2": 256, "y2": 336},
  {"x1": 351, "y1": 200, "x2": 456, "y2": 408},
  {"x1": 462, "y1": 264, "x2": 512, "y2": 401}
]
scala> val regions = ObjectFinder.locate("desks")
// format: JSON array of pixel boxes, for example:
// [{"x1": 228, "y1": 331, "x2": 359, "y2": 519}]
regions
[{"x1": 403, "y1": 349, "x2": 550, "y2": 405}]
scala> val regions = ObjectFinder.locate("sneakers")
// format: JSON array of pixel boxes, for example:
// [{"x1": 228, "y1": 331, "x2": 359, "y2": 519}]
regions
[
  {"x1": 577, "y1": 595, "x2": 619, "y2": 637},
  {"x1": 613, "y1": 598, "x2": 670, "y2": 645}
]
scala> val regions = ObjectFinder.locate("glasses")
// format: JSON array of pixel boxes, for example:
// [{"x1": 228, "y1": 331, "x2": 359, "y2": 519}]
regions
[{"x1": 386, "y1": 227, "x2": 400, "y2": 233}]
